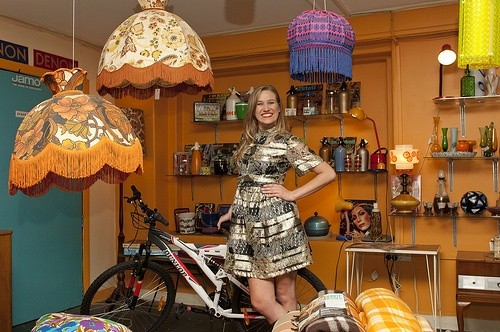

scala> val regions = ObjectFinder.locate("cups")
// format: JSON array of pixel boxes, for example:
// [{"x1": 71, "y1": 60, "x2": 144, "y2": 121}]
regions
[
  {"x1": 214, "y1": 157, "x2": 227, "y2": 175},
  {"x1": 235, "y1": 100, "x2": 249, "y2": 120}
]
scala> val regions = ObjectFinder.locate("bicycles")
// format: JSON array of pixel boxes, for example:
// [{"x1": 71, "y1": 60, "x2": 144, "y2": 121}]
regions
[{"x1": 79, "y1": 185, "x2": 327, "y2": 332}]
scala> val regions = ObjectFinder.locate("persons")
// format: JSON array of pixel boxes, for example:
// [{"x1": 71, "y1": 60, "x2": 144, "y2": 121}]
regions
[
  {"x1": 217, "y1": 85, "x2": 338, "y2": 329},
  {"x1": 346, "y1": 201, "x2": 373, "y2": 235}
]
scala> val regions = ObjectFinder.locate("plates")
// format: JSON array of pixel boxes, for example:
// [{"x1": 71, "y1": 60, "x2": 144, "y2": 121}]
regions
[{"x1": 430, "y1": 152, "x2": 477, "y2": 158}]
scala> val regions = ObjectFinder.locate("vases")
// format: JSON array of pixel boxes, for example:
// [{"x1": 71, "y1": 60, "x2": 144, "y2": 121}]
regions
[{"x1": 430, "y1": 116, "x2": 499, "y2": 157}]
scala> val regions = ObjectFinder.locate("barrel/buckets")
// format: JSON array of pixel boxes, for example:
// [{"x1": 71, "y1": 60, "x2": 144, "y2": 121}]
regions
[
  {"x1": 176, "y1": 213, "x2": 197, "y2": 234},
  {"x1": 370, "y1": 147, "x2": 388, "y2": 169}
]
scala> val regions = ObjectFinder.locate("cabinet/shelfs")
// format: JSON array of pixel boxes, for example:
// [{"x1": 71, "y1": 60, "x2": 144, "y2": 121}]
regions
[
  {"x1": 390, "y1": 96, "x2": 500, "y2": 247},
  {"x1": 166, "y1": 114, "x2": 378, "y2": 242},
  {"x1": 456, "y1": 251, "x2": 500, "y2": 332}
]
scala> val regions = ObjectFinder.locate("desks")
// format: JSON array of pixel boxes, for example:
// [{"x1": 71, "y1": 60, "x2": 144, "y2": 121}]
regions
[{"x1": 344, "y1": 244, "x2": 442, "y2": 332}]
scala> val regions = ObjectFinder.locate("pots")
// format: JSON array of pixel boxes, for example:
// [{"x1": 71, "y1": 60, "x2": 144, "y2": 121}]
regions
[{"x1": 304, "y1": 211, "x2": 331, "y2": 237}]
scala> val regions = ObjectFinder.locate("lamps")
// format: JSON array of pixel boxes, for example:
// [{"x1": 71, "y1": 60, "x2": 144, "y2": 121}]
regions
[
  {"x1": 457, "y1": 0, "x2": 500, "y2": 96},
  {"x1": 434, "y1": 44, "x2": 457, "y2": 99},
  {"x1": 390, "y1": 145, "x2": 420, "y2": 214},
  {"x1": 8, "y1": 0, "x2": 215, "y2": 197},
  {"x1": 287, "y1": 0, "x2": 355, "y2": 84}
]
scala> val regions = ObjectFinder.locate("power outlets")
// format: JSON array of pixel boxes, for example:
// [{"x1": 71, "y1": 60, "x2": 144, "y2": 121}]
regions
[{"x1": 386, "y1": 254, "x2": 412, "y2": 262}]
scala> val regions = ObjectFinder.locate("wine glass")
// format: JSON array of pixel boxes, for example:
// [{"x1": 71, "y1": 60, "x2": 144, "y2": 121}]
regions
[
  {"x1": 479, "y1": 126, "x2": 497, "y2": 157},
  {"x1": 423, "y1": 201, "x2": 460, "y2": 216}
]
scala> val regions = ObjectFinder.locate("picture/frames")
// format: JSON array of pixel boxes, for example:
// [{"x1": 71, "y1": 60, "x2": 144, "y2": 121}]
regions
[{"x1": 339, "y1": 199, "x2": 382, "y2": 242}]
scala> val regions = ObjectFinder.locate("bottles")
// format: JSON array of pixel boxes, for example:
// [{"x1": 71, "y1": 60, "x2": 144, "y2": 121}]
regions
[
  {"x1": 430, "y1": 116, "x2": 441, "y2": 152},
  {"x1": 326, "y1": 91, "x2": 337, "y2": 115},
  {"x1": 190, "y1": 141, "x2": 201, "y2": 176},
  {"x1": 441, "y1": 127, "x2": 449, "y2": 152},
  {"x1": 370, "y1": 203, "x2": 382, "y2": 240},
  {"x1": 434, "y1": 169, "x2": 450, "y2": 214},
  {"x1": 456, "y1": 140, "x2": 469, "y2": 151},
  {"x1": 304, "y1": 91, "x2": 312, "y2": 107},
  {"x1": 451, "y1": 127, "x2": 458, "y2": 152}
]
